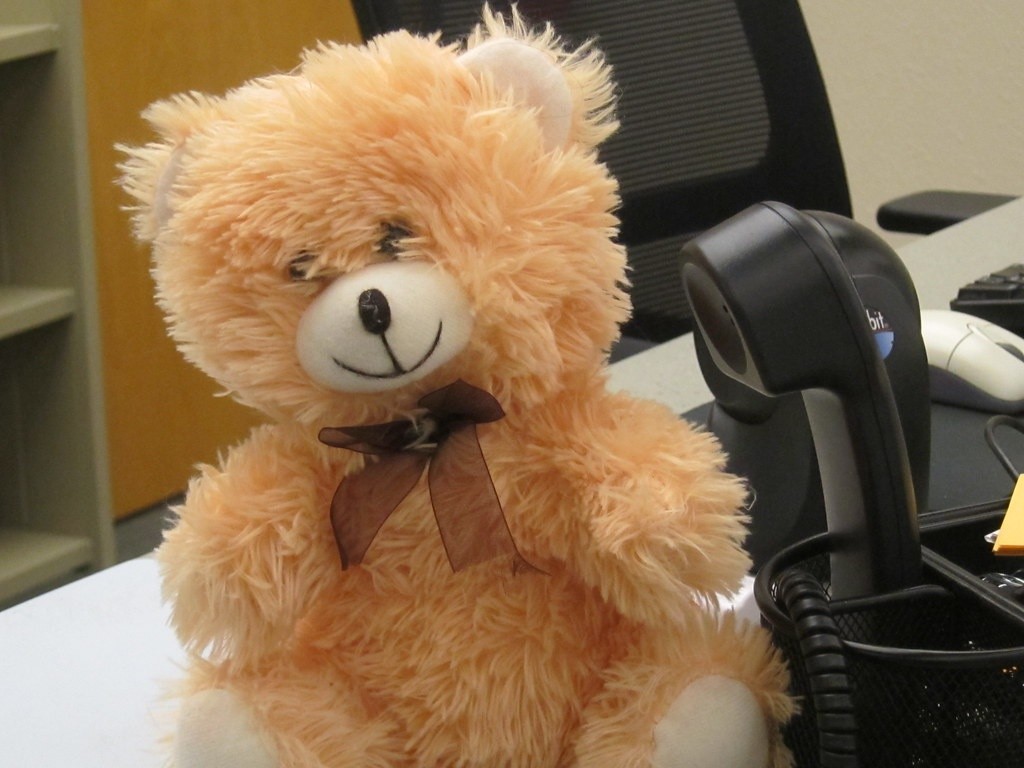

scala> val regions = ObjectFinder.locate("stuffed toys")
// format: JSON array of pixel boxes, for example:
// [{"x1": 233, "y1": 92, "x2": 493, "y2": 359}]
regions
[{"x1": 112, "y1": 0, "x2": 802, "y2": 768}]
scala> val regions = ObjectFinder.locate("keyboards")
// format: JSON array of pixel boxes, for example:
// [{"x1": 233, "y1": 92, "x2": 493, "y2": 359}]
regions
[{"x1": 949, "y1": 264, "x2": 1024, "y2": 335}]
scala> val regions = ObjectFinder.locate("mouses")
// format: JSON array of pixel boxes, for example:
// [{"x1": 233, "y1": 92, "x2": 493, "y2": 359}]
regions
[{"x1": 919, "y1": 308, "x2": 1024, "y2": 417}]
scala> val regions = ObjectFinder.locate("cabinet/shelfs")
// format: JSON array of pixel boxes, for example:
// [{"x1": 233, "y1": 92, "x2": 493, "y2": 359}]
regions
[{"x1": 0, "y1": 1, "x2": 118, "y2": 607}]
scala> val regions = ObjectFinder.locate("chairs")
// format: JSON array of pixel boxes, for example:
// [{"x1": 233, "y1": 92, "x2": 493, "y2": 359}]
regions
[{"x1": 346, "y1": 1, "x2": 857, "y2": 364}]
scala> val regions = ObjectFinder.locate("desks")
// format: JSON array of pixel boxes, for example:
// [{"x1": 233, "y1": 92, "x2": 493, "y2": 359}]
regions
[{"x1": 0, "y1": 199, "x2": 1023, "y2": 768}]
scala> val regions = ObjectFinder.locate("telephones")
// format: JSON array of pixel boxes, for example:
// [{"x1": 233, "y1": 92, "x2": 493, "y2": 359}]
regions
[{"x1": 677, "y1": 198, "x2": 931, "y2": 598}]
